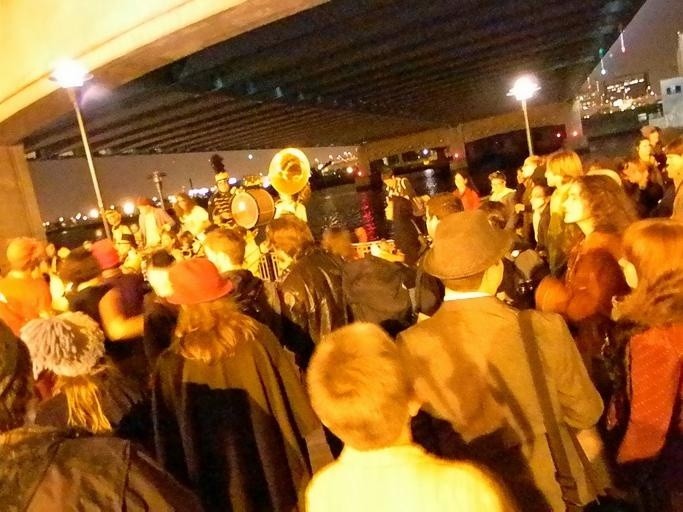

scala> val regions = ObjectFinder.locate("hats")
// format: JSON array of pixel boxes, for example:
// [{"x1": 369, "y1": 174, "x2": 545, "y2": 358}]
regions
[
  {"x1": 103, "y1": 209, "x2": 117, "y2": 220},
  {"x1": 214, "y1": 171, "x2": 228, "y2": 183},
  {"x1": 92, "y1": 242, "x2": 119, "y2": 272},
  {"x1": 423, "y1": 209, "x2": 513, "y2": 280},
  {"x1": 135, "y1": 196, "x2": 151, "y2": 206},
  {"x1": 165, "y1": 256, "x2": 235, "y2": 305}
]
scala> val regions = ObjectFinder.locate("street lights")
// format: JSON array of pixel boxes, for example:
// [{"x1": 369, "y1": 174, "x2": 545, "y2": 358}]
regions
[
  {"x1": 49, "y1": 62, "x2": 112, "y2": 238},
  {"x1": 147, "y1": 171, "x2": 166, "y2": 209},
  {"x1": 506, "y1": 77, "x2": 542, "y2": 156}
]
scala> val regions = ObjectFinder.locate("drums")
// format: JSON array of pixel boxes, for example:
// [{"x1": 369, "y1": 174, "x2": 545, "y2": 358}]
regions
[{"x1": 230, "y1": 189, "x2": 276, "y2": 230}]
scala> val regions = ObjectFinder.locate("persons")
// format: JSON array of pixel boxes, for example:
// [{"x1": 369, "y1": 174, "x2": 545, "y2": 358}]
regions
[
  {"x1": 392, "y1": 211, "x2": 620, "y2": 510},
  {"x1": 150, "y1": 258, "x2": 336, "y2": 510},
  {"x1": 599, "y1": 218, "x2": 681, "y2": 512},
  {"x1": 301, "y1": 321, "x2": 512, "y2": 510},
  {"x1": 0, "y1": 325, "x2": 200, "y2": 510},
  {"x1": 0, "y1": 127, "x2": 683, "y2": 454}
]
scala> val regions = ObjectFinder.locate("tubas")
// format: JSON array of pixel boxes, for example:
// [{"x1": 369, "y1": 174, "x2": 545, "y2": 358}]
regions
[{"x1": 269, "y1": 147, "x2": 311, "y2": 209}]
